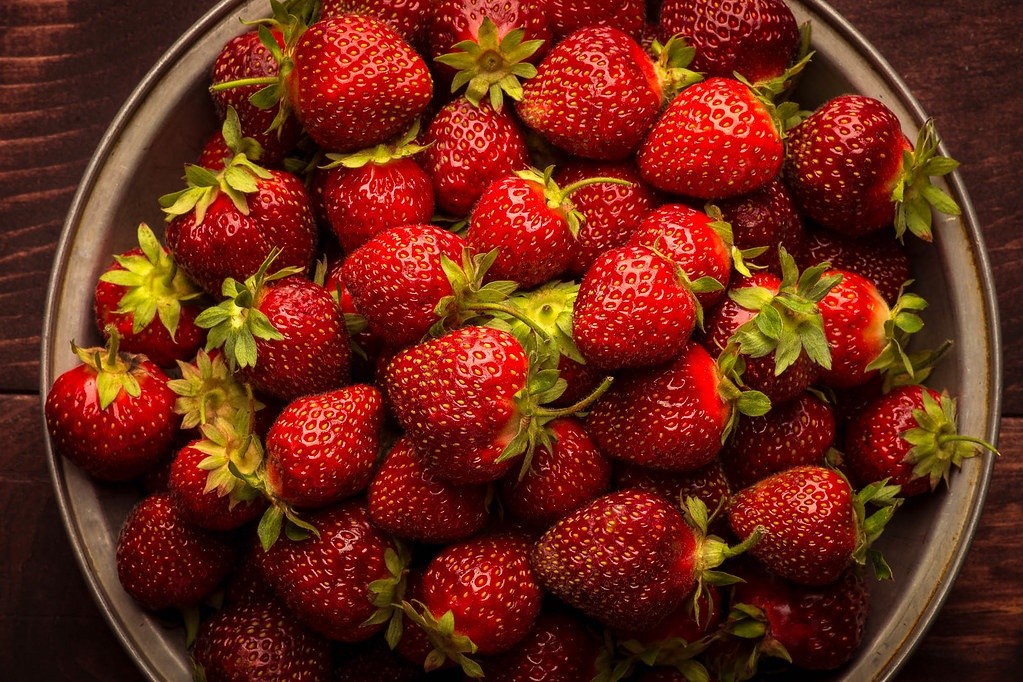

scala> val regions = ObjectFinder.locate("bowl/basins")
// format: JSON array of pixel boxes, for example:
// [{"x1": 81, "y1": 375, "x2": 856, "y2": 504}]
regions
[{"x1": 35, "y1": 1, "x2": 997, "y2": 682}]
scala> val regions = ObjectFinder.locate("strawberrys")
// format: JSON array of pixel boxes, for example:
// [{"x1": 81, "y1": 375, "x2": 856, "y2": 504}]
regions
[{"x1": 47, "y1": 0, "x2": 1002, "y2": 682}]
